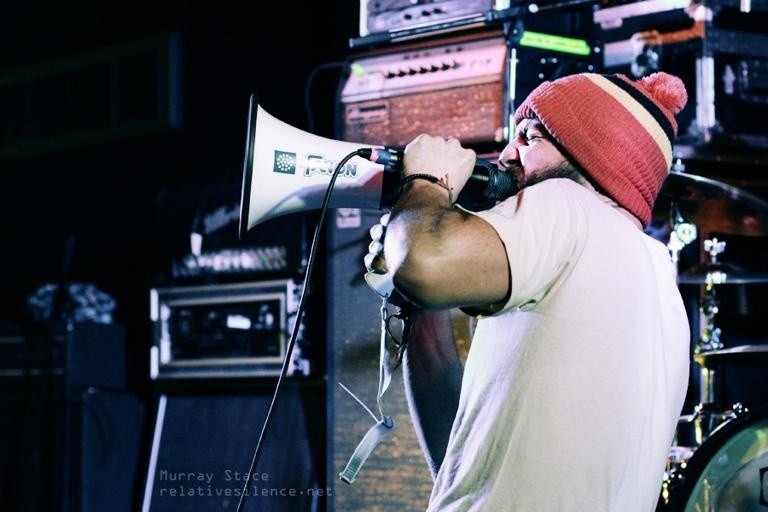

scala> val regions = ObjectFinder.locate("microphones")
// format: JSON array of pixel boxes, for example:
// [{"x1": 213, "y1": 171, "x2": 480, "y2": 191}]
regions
[{"x1": 362, "y1": 146, "x2": 514, "y2": 200}]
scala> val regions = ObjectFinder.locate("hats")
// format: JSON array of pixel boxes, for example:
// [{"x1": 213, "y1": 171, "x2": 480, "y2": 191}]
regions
[{"x1": 513, "y1": 66, "x2": 692, "y2": 232}]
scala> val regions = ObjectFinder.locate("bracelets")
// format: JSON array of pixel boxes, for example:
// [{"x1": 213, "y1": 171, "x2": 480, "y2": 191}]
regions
[{"x1": 397, "y1": 173, "x2": 452, "y2": 201}]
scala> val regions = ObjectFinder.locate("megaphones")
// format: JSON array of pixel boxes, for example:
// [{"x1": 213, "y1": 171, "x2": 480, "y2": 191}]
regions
[{"x1": 237, "y1": 92, "x2": 498, "y2": 240}]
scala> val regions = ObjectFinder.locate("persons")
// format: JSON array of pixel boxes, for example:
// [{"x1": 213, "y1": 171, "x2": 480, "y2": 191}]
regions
[{"x1": 364, "y1": 71, "x2": 692, "y2": 512}]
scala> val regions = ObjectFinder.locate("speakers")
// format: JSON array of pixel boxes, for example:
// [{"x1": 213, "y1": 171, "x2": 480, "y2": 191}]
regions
[{"x1": 50, "y1": 316, "x2": 151, "y2": 511}]
